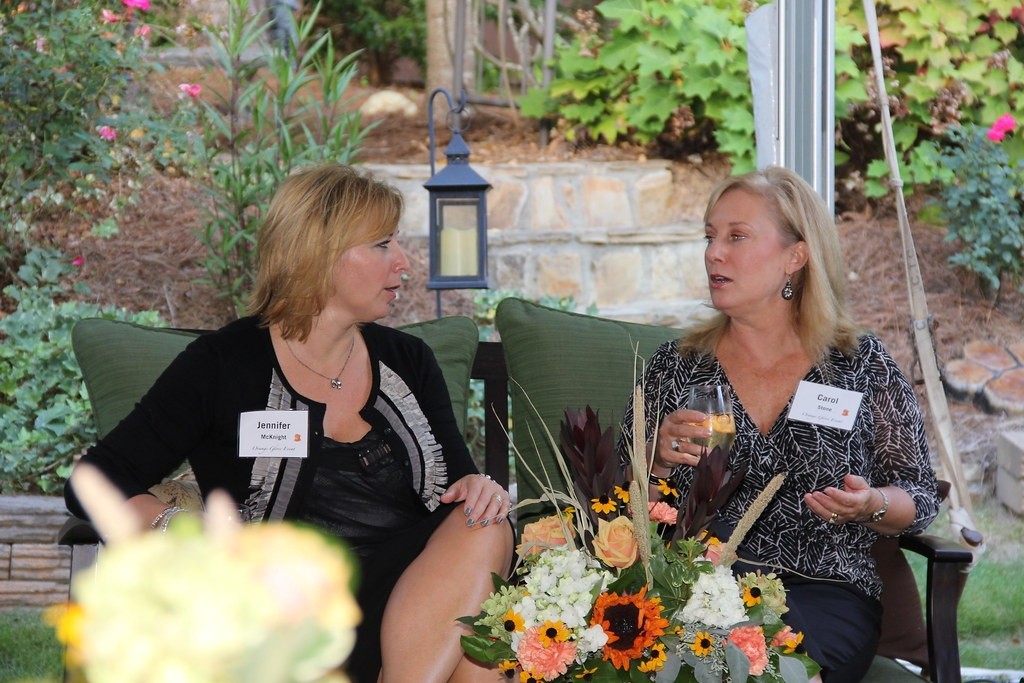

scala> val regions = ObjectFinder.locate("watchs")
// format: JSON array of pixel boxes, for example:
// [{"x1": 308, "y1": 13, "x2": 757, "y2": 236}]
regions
[{"x1": 859, "y1": 487, "x2": 888, "y2": 524}]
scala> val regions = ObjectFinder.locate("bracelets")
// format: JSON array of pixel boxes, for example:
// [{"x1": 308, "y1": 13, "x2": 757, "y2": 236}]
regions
[
  {"x1": 150, "y1": 506, "x2": 190, "y2": 531},
  {"x1": 646, "y1": 466, "x2": 679, "y2": 485},
  {"x1": 476, "y1": 473, "x2": 499, "y2": 485}
]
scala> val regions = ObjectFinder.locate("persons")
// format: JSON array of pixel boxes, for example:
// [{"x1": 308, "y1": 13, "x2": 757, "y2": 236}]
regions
[
  {"x1": 61, "y1": 161, "x2": 518, "y2": 683},
  {"x1": 602, "y1": 165, "x2": 940, "y2": 683}
]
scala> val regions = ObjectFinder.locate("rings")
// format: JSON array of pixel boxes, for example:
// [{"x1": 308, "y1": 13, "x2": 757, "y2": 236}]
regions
[
  {"x1": 827, "y1": 512, "x2": 838, "y2": 524},
  {"x1": 492, "y1": 494, "x2": 503, "y2": 505},
  {"x1": 672, "y1": 439, "x2": 682, "y2": 452}
]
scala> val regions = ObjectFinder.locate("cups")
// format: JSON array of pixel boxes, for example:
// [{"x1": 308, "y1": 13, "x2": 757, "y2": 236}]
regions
[{"x1": 688, "y1": 386, "x2": 735, "y2": 466}]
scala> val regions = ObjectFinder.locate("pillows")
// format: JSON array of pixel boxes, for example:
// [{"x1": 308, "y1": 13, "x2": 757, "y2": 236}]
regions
[
  {"x1": 71, "y1": 317, "x2": 481, "y2": 480},
  {"x1": 496, "y1": 297, "x2": 702, "y2": 573}
]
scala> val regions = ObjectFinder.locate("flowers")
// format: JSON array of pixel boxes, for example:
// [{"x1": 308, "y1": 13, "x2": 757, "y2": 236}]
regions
[
  {"x1": 47, "y1": 465, "x2": 365, "y2": 681},
  {"x1": 453, "y1": 332, "x2": 829, "y2": 683}
]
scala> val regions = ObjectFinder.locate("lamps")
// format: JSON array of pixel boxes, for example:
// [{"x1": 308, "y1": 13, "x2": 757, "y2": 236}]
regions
[{"x1": 425, "y1": 107, "x2": 494, "y2": 291}]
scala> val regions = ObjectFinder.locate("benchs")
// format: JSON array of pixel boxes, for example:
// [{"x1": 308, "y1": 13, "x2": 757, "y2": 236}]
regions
[{"x1": 56, "y1": 326, "x2": 974, "y2": 683}]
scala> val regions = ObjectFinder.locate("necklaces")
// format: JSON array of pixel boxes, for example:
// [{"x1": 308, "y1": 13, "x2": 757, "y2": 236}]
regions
[{"x1": 277, "y1": 317, "x2": 355, "y2": 389}]
